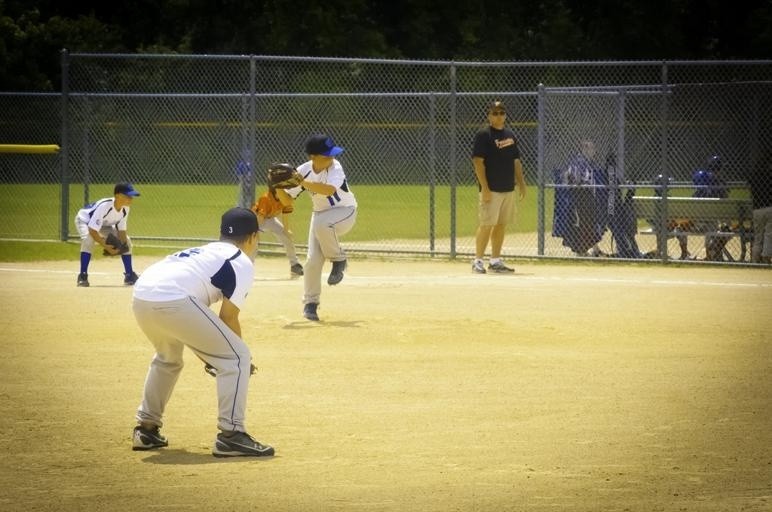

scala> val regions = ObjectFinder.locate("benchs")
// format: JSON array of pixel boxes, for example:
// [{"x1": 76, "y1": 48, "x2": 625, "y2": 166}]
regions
[{"x1": 629, "y1": 196, "x2": 753, "y2": 262}]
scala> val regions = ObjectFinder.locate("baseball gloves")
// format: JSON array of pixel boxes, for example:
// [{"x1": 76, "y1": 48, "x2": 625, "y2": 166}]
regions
[
  {"x1": 103, "y1": 233, "x2": 129, "y2": 256},
  {"x1": 270, "y1": 163, "x2": 300, "y2": 189}
]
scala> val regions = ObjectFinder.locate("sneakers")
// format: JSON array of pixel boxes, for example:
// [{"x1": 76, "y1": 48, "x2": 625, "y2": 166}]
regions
[
  {"x1": 472, "y1": 261, "x2": 486, "y2": 273},
  {"x1": 212, "y1": 432, "x2": 274, "y2": 457},
  {"x1": 488, "y1": 262, "x2": 514, "y2": 272},
  {"x1": 328, "y1": 259, "x2": 346, "y2": 284},
  {"x1": 303, "y1": 302, "x2": 319, "y2": 320},
  {"x1": 575, "y1": 249, "x2": 609, "y2": 257},
  {"x1": 125, "y1": 272, "x2": 138, "y2": 282},
  {"x1": 132, "y1": 427, "x2": 168, "y2": 449},
  {"x1": 291, "y1": 263, "x2": 303, "y2": 274},
  {"x1": 78, "y1": 272, "x2": 89, "y2": 286}
]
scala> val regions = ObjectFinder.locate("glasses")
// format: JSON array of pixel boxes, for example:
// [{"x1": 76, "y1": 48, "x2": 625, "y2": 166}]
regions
[{"x1": 493, "y1": 111, "x2": 507, "y2": 115}]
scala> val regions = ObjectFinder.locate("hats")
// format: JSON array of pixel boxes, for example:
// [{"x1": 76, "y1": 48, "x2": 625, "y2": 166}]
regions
[
  {"x1": 115, "y1": 184, "x2": 140, "y2": 196},
  {"x1": 490, "y1": 101, "x2": 507, "y2": 111},
  {"x1": 305, "y1": 135, "x2": 344, "y2": 156},
  {"x1": 222, "y1": 208, "x2": 264, "y2": 237}
]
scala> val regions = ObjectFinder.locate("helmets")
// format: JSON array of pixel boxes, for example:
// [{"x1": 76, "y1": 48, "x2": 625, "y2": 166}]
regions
[
  {"x1": 692, "y1": 155, "x2": 730, "y2": 197},
  {"x1": 655, "y1": 175, "x2": 671, "y2": 184}
]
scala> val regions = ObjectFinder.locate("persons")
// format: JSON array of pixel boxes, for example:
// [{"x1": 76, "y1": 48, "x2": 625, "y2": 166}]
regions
[
  {"x1": 73, "y1": 182, "x2": 140, "y2": 288},
  {"x1": 555, "y1": 140, "x2": 606, "y2": 256},
  {"x1": 237, "y1": 149, "x2": 253, "y2": 209},
  {"x1": 470, "y1": 100, "x2": 527, "y2": 275},
  {"x1": 252, "y1": 191, "x2": 304, "y2": 276},
  {"x1": 605, "y1": 149, "x2": 772, "y2": 263},
  {"x1": 269, "y1": 133, "x2": 358, "y2": 322},
  {"x1": 131, "y1": 206, "x2": 275, "y2": 458}
]
